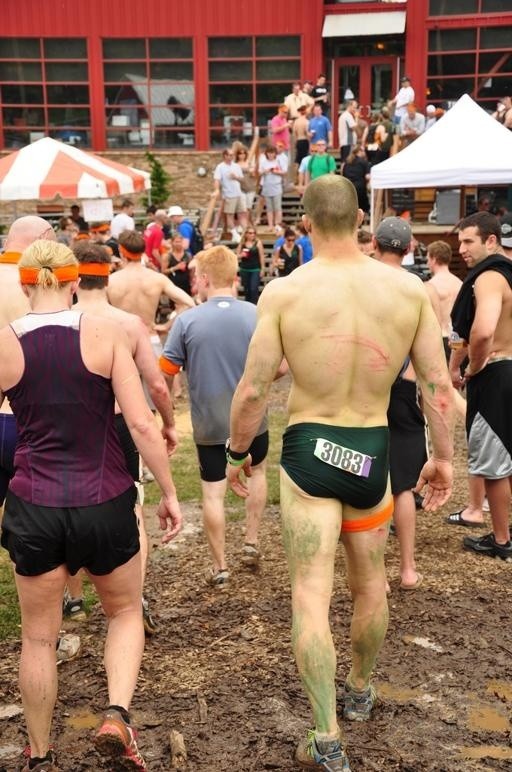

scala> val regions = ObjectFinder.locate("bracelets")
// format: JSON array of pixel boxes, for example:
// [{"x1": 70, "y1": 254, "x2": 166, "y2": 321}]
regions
[{"x1": 224, "y1": 454, "x2": 249, "y2": 467}]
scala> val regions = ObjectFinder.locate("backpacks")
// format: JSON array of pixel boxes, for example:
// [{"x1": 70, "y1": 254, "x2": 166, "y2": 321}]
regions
[{"x1": 183, "y1": 221, "x2": 204, "y2": 257}]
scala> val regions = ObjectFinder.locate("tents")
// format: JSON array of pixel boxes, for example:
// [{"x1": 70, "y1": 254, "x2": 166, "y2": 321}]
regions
[
  {"x1": 1, "y1": 135, "x2": 150, "y2": 217},
  {"x1": 372, "y1": 93, "x2": 512, "y2": 234}
]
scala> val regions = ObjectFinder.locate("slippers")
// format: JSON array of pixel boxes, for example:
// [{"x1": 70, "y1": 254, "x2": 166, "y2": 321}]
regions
[
  {"x1": 444, "y1": 508, "x2": 486, "y2": 528},
  {"x1": 399, "y1": 572, "x2": 423, "y2": 590}
]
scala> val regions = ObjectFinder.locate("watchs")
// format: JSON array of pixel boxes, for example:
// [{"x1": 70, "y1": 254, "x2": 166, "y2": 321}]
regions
[
  {"x1": 61, "y1": 241, "x2": 178, "y2": 636},
  {"x1": 222, "y1": 436, "x2": 251, "y2": 461}
]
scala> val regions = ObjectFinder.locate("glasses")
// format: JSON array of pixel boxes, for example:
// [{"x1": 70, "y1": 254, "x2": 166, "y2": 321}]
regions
[
  {"x1": 288, "y1": 239, "x2": 294, "y2": 241},
  {"x1": 246, "y1": 232, "x2": 256, "y2": 234}
]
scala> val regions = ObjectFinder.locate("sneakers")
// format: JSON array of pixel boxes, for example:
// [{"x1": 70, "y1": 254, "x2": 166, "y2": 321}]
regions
[
  {"x1": 94, "y1": 709, "x2": 146, "y2": 771},
  {"x1": 53, "y1": 632, "x2": 80, "y2": 664},
  {"x1": 141, "y1": 598, "x2": 159, "y2": 634},
  {"x1": 342, "y1": 677, "x2": 377, "y2": 722},
  {"x1": 462, "y1": 532, "x2": 512, "y2": 562},
  {"x1": 19, "y1": 759, "x2": 55, "y2": 772},
  {"x1": 238, "y1": 546, "x2": 260, "y2": 568},
  {"x1": 62, "y1": 593, "x2": 87, "y2": 622},
  {"x1": 204, "y1": 568, "x2": 230, "y2": 588},
  {"x1": 295, "y1": 736, "x2": 349, "y2": 772}
]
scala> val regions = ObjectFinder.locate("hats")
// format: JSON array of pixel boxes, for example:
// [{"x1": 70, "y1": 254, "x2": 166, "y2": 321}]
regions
[
  {"x1": 167, "y1": 205, "x2": 184, "y2": 217},
  {"x1": 375, "y1": 215, "x2": 411, "y2": 249}
]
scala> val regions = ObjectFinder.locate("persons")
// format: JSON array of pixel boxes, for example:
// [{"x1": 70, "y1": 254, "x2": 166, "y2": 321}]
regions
[
  {"x1": 385, "y1": 76, "x2": 414, "y2": 123},
  {"x1": 476, "y1": 196, "x2": 490, "y2": 213},
  {"x1": 55, "y1": 216, "x2": 74, "y2": 248},
  {"x1": 209, "y1": 147, "x2": 250, "y2": 231},
  {"x1": 0, "y1": 215, "x2": 60, "y2": 512},
  {"x1": 109, "y1": 201, "x2": 136, "y2": 237},
  {"x1": 234, "y1": 125, "x2": 257, "y2": 235},
  {"x1": 235, "y1": 226, "x2": 264, "y2": 305},
  {"x1": 301, "y1": 79, "x2": 313, "y2": 95},
  {"x1": 167, "y1": 205, "x2": 204, "y2": 256},
  {"x1": 68, "y1": 204, "x2": 88, "y2": 236},
  {"x1": 283, "y1": 81, "x2": 315, "y2": 120},
  {"x1": 256, "y1": 145, "x2": 288, "y2": 232},
  {"x1": 226, "y1": 174, "x2": 459, "y2": 770},
  {"x1": 292, "y1": 105, "x2": 310, "y2": 185},
  {"x1": 304, "y1": 139, "x2": 336, "y2": 187},
  {"x1": 297, "y1": 143, "x2": 318, "y2": 195},
  {"x1": 158, "y1": 245, "x2": 288, "y2": 592},
  {"x1": 104, "y1": 229, "x2": 202, "y2": 476},
  {"x1": 273, "y1": 222, "x2": 285, "y2": 247},
  {"x1": 374, "y1": 216, "x2": 430, "y2": 591},
  {"x1": 309, "y1": 72, "x2": 329, "y2": 117},
  {"x1": 161, "y1": 234, "x2": 194, "y2": 301},
  {"x1": 0, "y1": 239, "x2": 183, "y2": 771},
  {"x1": 422, "y1": 241, "x2": 470, "y2": 394},
  {"x1": 272, "y1": 226, "x2": 302, "y2": 278},
  {"x1": 275, "y1": 140, "x2": 288, "y2": 168},
  {"x1": 489, "y1": 96, "x2": 512, "y2": 131},
  {"x1": 296, "y1": 222, "x2": 313, "y2": 265},
  {"x1": 270, "y1": 105, "x2": 291, "y2": 159},
  {"x1": 361, "y1": 111, "x2": 380, "y2": 163},
  {"x1": 355, "y1": 118, "x2": 370, "y2": 163},
  {"x1": 337, "y1": 99, "x2": 359, "y2": 162},
  {"x1": 143, "y1": 210, "x2": 167, "y2": 272},
  {"x1": 342, "y1": 144, "x2": 372, "y2": 220},
  {"x1": 425, "y1": 104, "x2": 437, "y2": 131},
  {"x1": 306, "y1": 105, "x2": 333, "y2": 153},
  {"x1": 445, "y1": 211, "x2": 512, "y2": 561},
  {"x1": 396, "y1": 100, "x2": 425, "y2": 146},
  {"x1": 374, "y1": 106, "x2": 400, "y2": 161},
  {"x1": 444, "y1": 476, "x2": 488, "y2": 528}
]
